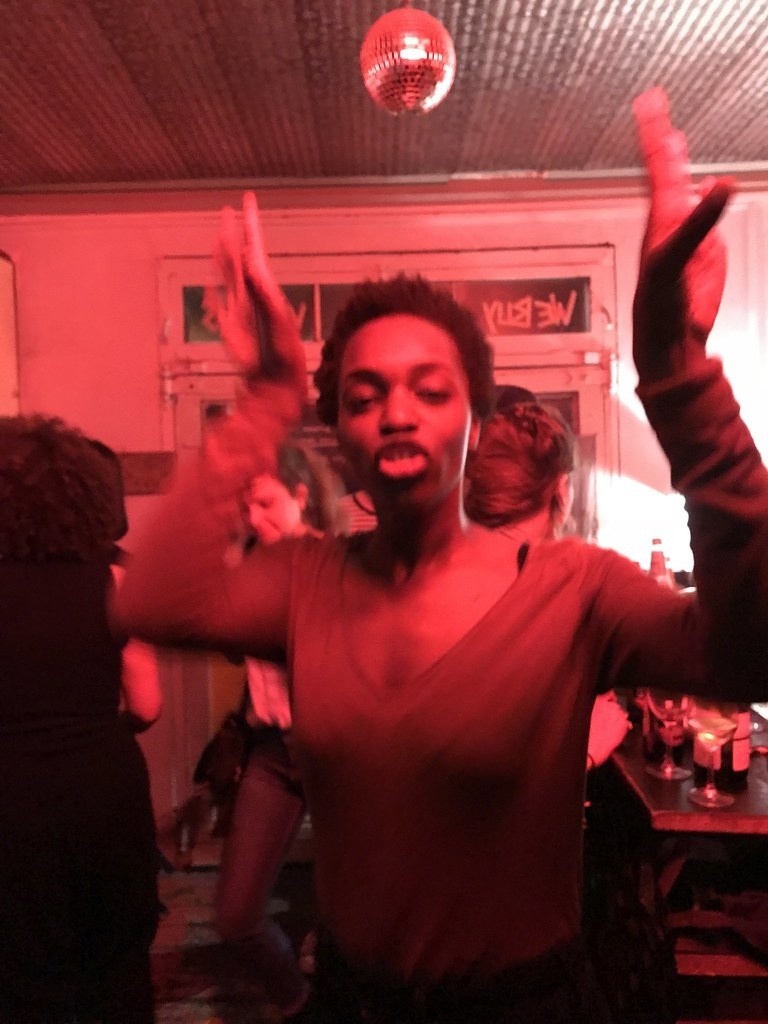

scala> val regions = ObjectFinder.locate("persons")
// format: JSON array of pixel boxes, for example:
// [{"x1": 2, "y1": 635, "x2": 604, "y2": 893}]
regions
[
  {"x1": 0, "y1": 413, "x2": 171, "y2": 1024},
  {"x1": 108, "y1": 86, "x2": 768, "y2": 1024}
]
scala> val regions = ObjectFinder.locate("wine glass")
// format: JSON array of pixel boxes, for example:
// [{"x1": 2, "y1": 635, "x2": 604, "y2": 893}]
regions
[
  {"x1": 647, "y1": 687, "x2": 693, "y2": 779},
  {"x1": 686, "y1": 696, "x2": 737, "y2": 805}
]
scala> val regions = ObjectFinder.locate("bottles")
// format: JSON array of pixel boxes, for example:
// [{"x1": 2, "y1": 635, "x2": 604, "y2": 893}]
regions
[
  {"x1": 665, "y1": 557, "x2": 677, "y2": 592},
  {"x1": 650, "y1": 538, "x2": 667, "y2": 588},
  {"x1": 697, "y1": 698, "x2": 751, "y2": 791}
]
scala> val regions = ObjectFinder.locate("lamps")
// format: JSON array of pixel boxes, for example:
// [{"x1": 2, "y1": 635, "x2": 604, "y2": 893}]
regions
[{"x1": 360, "y1": 1, "x2": 457, "y2": 119}]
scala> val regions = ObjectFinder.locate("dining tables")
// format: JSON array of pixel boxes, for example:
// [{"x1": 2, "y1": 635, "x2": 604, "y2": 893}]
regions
[{"x1": 607, "y1": 704, "x2": 768, "y2": 835}]
